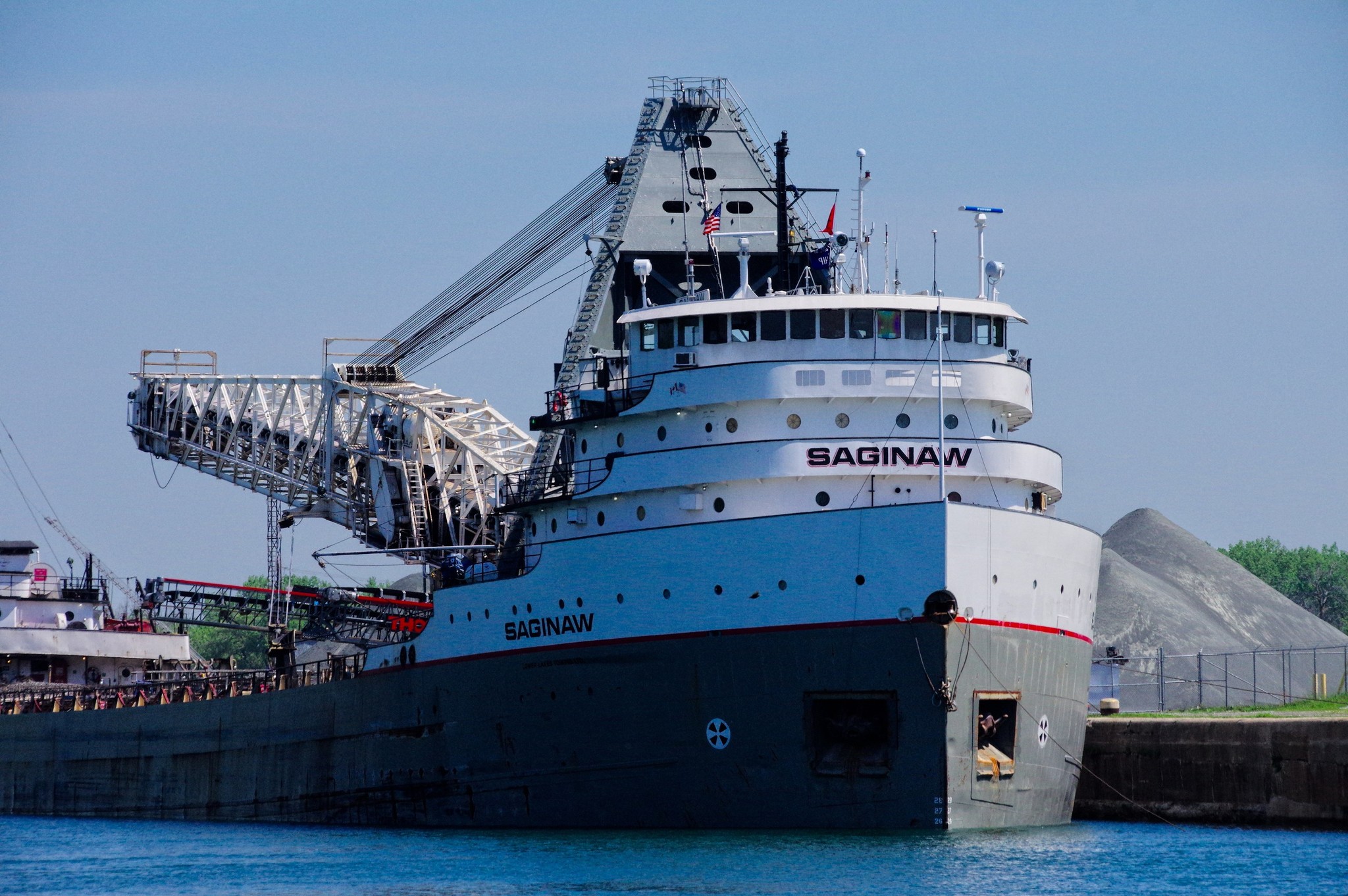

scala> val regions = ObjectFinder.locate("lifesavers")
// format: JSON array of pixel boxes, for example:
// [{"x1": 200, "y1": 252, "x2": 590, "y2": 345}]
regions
[{"x1": 553, "y1": 391, "x2": 563, "y2": 414}]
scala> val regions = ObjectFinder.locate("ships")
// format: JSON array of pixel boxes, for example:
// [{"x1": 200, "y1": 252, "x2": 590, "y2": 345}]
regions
[{"x1": 0, "y1": 75, "x2": 1101, "y2": 834}]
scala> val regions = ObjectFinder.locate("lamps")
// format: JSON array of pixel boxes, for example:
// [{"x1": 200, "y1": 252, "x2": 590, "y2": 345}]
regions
[
  {"x1": 829, "y1": 231, "x2": 849, "y2": 253},
  {"x1": 1031, "y1": 492, "x2": 1048, "y2": 511},
  {"x1": 985, "y1": 261, "x2": 1005, "y2": 281},
  {"x1": 633, "y1": 258, "x2": 651, "y2": 278}
]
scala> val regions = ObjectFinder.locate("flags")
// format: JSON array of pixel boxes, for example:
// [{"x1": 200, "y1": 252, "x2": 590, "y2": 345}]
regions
[
  {"x1": 818, "y1": 203, "x2": 835, "y2": 236},
  {"x1": 703, "y1": 202, "x2": 720, "y2": 235}
]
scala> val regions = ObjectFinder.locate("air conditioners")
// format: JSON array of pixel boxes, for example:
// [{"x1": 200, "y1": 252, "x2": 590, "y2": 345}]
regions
[{"x1": 674, "y1": 352, "x2": 696, "y2": 365}]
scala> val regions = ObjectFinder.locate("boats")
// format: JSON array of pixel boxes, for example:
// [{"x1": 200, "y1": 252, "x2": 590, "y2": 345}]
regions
[{"x1": 0, "y1": 682, "x2": 242, "y2": 715}]
